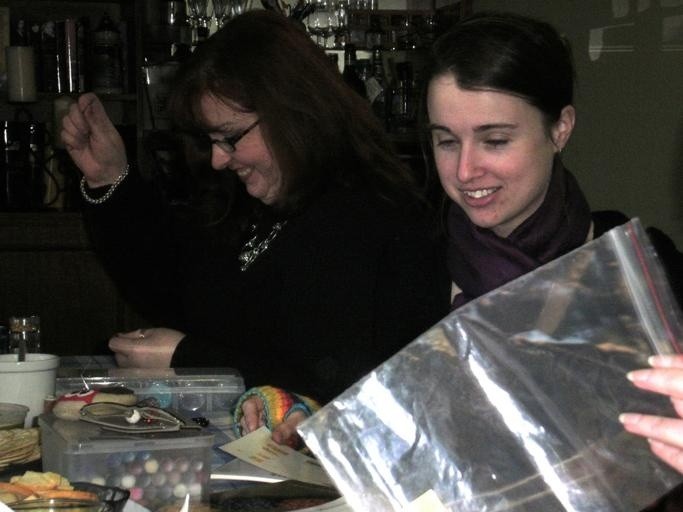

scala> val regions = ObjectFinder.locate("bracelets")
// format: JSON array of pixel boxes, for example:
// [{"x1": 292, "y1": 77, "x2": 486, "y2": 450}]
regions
[{"x1": 77, "y1": 165, "x2": 131, "y2": 207}]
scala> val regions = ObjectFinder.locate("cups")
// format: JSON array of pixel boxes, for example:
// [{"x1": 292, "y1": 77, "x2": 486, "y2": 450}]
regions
[{"x1": 8, "y1": 313, "x2": 41, "y2": 353}]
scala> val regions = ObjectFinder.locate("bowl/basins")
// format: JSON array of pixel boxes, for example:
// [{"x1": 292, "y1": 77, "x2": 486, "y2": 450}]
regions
[{"x1": 0, "y1": 353, "x2": 59, "y2": 429}]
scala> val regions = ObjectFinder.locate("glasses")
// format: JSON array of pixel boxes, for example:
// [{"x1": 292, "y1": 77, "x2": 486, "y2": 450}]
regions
[
  {"x1": 78, "y1": 401, "x2": 186, "y2": 434},
  {"x1": 199, "y1": 118, "x2": 260, "y2": 154}
]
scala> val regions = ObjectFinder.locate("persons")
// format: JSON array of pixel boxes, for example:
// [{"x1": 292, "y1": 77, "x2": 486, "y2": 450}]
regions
[
  {"x1": 224, "y1": 6, "x2": 683, "y2": 512},
  {"x1": 56, "y1": 6, "x2": 439, "y2": 406}
]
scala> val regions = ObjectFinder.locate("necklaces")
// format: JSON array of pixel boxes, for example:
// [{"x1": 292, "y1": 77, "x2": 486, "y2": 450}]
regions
[{"x1": 234, "y1": 213, "x2": 291, "y2": 273}]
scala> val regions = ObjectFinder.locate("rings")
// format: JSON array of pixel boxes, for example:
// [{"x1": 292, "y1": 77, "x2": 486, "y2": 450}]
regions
[{"x1": 137, "y1": 327, "x2": 146, "y2": 338}]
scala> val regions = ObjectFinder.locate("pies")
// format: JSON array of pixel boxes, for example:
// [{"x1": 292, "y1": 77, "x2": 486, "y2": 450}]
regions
[{"x1": 0, "y1": 428, "x2": 39, "y2": 468}]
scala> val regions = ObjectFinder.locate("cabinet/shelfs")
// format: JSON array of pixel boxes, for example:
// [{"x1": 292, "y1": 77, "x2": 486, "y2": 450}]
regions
[{"x1": 0, "y1": 0, "x2": 472, "y2": 354}]
